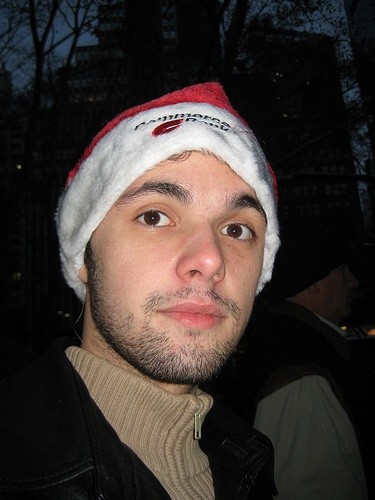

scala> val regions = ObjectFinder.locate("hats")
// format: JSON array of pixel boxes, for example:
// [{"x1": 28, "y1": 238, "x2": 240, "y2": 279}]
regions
[
  {"x1": 54, "y1": 80, "x2": 282, "y2": 298},
  {"x1": 262, "y1": 216, "x2": 348, "y2": 304}
]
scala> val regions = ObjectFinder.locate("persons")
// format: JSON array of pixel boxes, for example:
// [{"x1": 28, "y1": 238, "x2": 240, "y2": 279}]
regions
[
  {"x1": 0, "y1": 79, "x2": 281, "y2": 500},
  {"x1": 212, "y1": 218, "x2": 375, "y2": 500}
]
